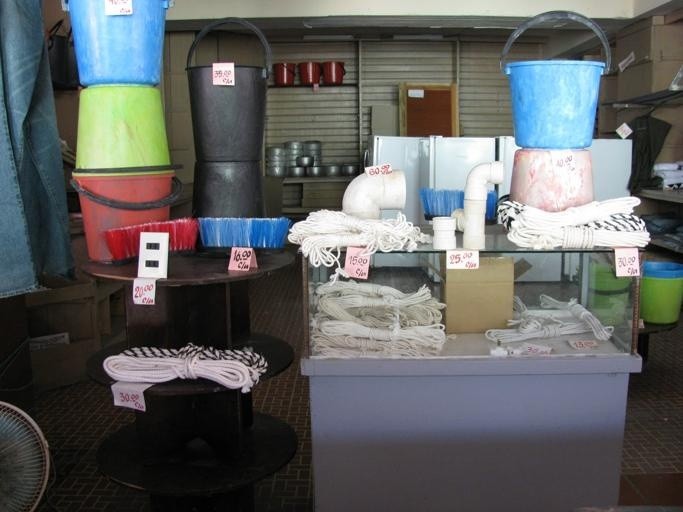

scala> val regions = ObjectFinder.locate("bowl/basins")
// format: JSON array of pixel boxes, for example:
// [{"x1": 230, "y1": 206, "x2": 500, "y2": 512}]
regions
[{"x1": 264, "y1": 140, "x2": 359, "y2": 176}]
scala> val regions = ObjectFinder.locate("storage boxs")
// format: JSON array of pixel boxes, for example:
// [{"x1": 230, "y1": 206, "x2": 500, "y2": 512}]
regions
[{"x1": 582, "y1": 24, "x2": 683, "y2": 165}]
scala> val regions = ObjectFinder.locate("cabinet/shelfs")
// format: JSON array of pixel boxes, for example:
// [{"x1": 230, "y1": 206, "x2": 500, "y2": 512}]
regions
[
  {"x1": 600, "y1": 89, "x2": 683, "y2": 255},
  {"x1": 263, "y1": 83, "x2": 363, "y2": 214}
]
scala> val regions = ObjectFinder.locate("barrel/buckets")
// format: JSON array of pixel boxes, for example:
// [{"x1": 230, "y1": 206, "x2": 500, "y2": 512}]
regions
[
  {"x1": 509, "y1": 149, "x2": 594, "y2": 212},
  {"x1": 500, "y1": 10, "x2": 612, "y2": 148},
  {"x1": 641, "y1": 261, "x2": 683, "y2": 278},
  {"x1": 61, "y1": 0, "x2": 173, "y2": 86},
  {"x1": 72, "y1": 84, "x2": 184, "y2": 176},
  {"x1": 573, "y1": 260, "x2": 633, "y2": 325},
  {"x1": 639, "y1": 278, "x2": 683, "y2": 324},
  {"x1": 70, "y1": 174, "x2": 184, "y2": 264},
  {"x1": 273, "y1": 63, "x2": 296, "y2": 85},
  {"x1": 322, "y1": 62, "x2": 346, "y2": 84},
  {"x1": 298, "y1": 62, "x2": 321, "y2": 84},
  {"x1": 190, "y1": 163, "x2": 264, "y2": 259},
  {"x1": 186, "y1": 17, "x2": 272, "y2": 163}
]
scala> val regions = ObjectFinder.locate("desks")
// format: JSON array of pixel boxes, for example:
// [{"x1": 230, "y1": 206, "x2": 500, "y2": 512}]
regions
[{"x1": 70, "y1": 236, "x2": 296, "y2": 494}]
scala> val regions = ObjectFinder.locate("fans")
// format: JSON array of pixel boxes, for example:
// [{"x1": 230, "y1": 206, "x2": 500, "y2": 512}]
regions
[{"x1": 0, "y1": 400, "x2": 50, "y2": 512}]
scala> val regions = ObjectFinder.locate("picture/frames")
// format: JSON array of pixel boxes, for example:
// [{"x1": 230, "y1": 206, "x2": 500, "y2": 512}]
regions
[{"x1": 398, "y1": 80, "x2": 460, "y2": 138}]
